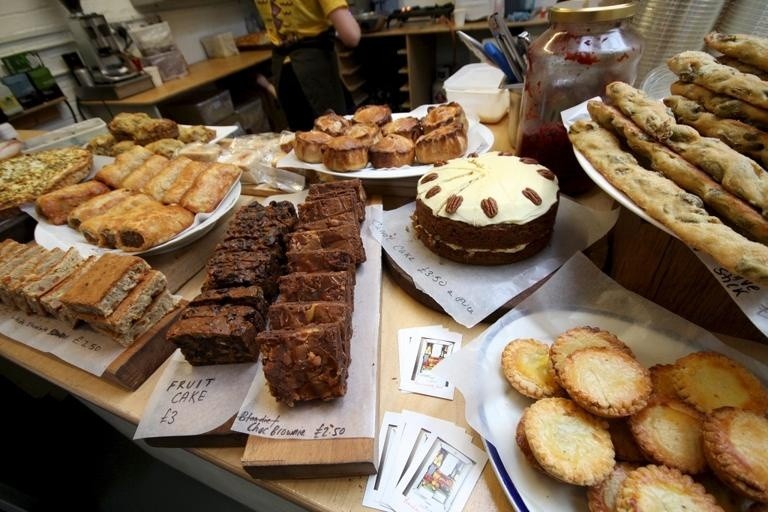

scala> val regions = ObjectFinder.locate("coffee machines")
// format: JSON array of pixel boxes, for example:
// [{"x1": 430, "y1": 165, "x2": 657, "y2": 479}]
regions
[{"x1": 55, "y1": 1, "x2": 138, "y2": 82}]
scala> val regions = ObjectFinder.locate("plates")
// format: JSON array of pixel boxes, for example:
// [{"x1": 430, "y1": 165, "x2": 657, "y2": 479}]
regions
[
  {"x1": 35, "y1": 181, "x2": 242, "y2": 258},
  {"x1": 470, "y1": 303, "x2": 767, "y2": 512},
  {"x1": 84, "y1": 123, "x2": 240, "y2": 156},
  {"x1": 560, "y1": 113, "x2": 685, "y2": 248},
  {"x1": 315, "y1": 111, "x2": 494, "y2": 180}
]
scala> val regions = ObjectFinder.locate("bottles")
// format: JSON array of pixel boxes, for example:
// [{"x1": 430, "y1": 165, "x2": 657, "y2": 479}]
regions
[{"x1": 72, "y1": 68, "x2": 94, "y2": 88}]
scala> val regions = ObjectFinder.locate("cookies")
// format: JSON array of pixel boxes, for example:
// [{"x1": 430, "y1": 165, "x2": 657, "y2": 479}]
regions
[{"x1": 502, "y1": 325, "x2": 768, "y2": 512}]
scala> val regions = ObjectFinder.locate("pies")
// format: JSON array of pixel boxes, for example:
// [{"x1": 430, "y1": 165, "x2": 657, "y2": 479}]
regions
[{"x1": 567, "y1": 31, "x2": 768, "y2": 294}]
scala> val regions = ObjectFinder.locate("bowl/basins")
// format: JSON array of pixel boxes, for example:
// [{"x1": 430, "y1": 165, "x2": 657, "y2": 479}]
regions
[{"x1": 356, "y1": 13, "x2": 388, "y2": 33}]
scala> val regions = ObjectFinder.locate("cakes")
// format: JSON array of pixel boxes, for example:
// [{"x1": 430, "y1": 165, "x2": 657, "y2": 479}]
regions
[{"x1": 413, "y1": 151, "x2": 560, "y2": 266}]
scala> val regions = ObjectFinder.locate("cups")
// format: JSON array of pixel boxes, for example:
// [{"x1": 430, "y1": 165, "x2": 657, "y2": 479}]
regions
[{"x1": 453, "y1": 9, "x2": 466, "y2": 28}]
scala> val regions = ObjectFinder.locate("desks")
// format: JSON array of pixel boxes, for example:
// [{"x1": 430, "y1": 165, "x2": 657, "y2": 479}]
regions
[{"x1": 0, "y1": 127, "x2": 532, "y2": 511}]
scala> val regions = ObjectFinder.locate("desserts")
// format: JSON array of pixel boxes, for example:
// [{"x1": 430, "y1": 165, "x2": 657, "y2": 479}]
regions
[{"x1": 0, "y1": 102, "x2": 468, "y2": 406}]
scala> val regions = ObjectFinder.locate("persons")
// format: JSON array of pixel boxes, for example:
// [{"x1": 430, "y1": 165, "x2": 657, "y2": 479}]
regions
[{"x1": 252, "y1": 1, "x2": 363, "y2": 132}]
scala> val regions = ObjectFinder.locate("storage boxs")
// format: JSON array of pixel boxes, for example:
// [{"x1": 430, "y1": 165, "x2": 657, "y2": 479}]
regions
[
  {"x1": 135, "y1": 47, "x2": 189, "y2": 80},
  {"x1": 441, "y1": 61, "x2": 512, "y2": 126},
  {"x1": 184, "y1": 91, "x2": 270, "y2": 133},
  {"x1": 126, "y1": 20, "x2": 174, "y2": 56}
]
